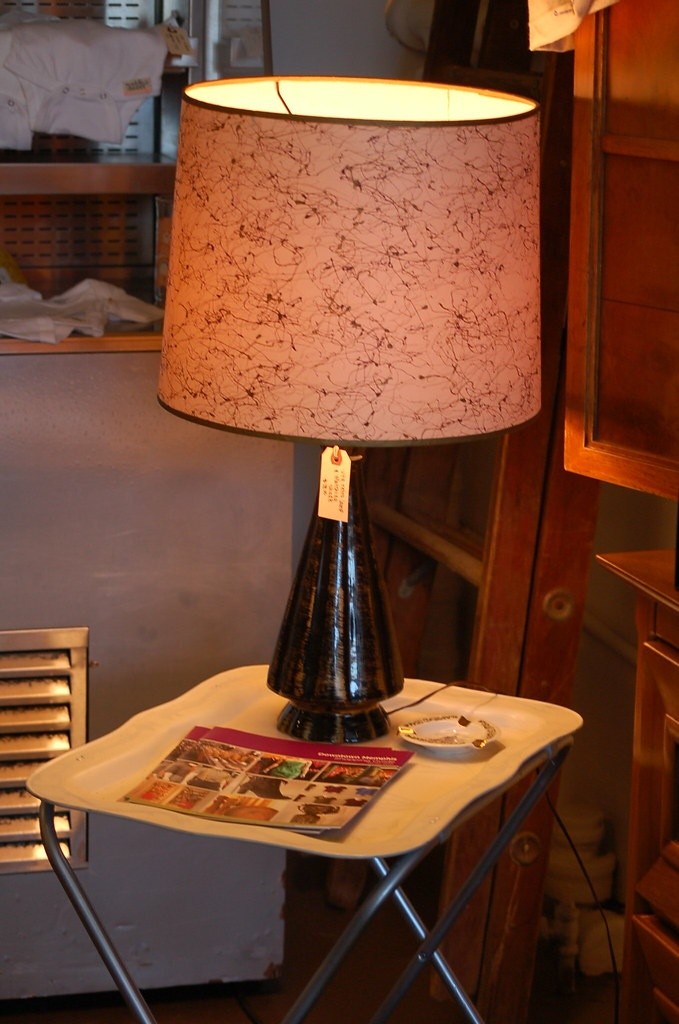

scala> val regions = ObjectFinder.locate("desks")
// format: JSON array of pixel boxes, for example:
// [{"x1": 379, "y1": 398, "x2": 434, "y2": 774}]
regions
[{"x1": 24, "y1": 663, "x2": 584, "y2": 1024}]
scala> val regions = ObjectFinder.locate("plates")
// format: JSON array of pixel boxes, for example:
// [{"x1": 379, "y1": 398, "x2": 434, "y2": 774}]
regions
[{"x1": 398, "y1": 716, "x2": 500, "y2": 755}]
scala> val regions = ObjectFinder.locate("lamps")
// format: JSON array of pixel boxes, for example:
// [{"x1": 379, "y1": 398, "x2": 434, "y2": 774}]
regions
[{"x1": 158, "y1": 76, "x2": 542, "y2": 742}]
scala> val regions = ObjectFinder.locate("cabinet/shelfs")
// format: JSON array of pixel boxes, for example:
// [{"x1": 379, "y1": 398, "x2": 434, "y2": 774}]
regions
[
  {"x1": 463, "y1": 0, "x2": 679, "y2": 1024},
  {"x1": 1, "y1": 0, "x2": 274, "y2": 354}
]
